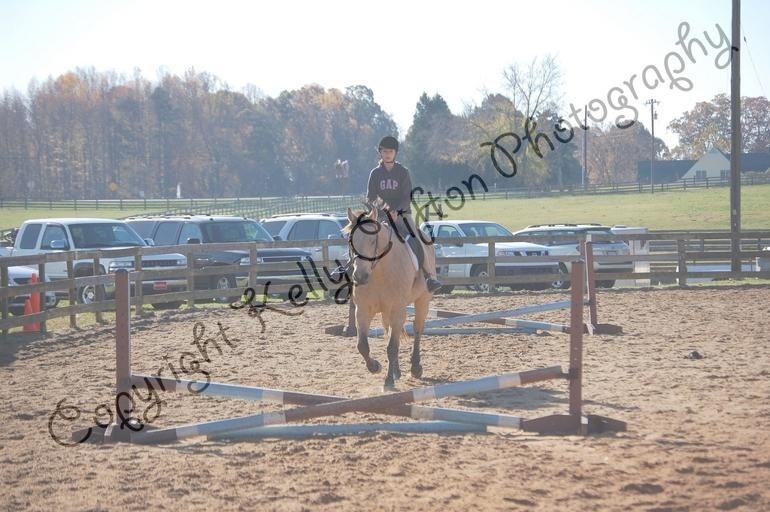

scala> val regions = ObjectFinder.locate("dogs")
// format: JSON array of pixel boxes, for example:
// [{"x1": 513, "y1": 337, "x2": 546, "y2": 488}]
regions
[{"x1": 339, "y1": 201, "x2": 438, "y2": 394}]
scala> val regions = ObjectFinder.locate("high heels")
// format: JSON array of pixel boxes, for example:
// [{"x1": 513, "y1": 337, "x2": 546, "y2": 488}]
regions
[{"x1": 22, "y1": 270, "x2": 42, "y2": 332}]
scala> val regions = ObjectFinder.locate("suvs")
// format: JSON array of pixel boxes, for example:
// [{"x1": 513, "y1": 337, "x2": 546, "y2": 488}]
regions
[{"x1": 0, "y1": 220, "x2": 632, "y2": 316}]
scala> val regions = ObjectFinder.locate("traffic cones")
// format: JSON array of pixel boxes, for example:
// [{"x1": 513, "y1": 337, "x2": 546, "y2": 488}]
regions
[{"x1": 22, "y1": 270, "x2": 42, "y2": 332}]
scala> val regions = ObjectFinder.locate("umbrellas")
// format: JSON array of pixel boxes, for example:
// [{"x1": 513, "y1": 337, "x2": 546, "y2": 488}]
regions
[{"x1": 427, "y1": 276, "x2": 441, "y2": 293}]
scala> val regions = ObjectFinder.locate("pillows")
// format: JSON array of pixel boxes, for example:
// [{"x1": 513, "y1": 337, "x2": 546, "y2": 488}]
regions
[{"x1": 378, "y1": 136, "x2": 398, "y2": 151}]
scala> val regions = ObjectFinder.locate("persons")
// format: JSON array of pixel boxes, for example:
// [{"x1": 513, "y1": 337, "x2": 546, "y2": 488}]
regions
[{"x1": 332, "y1": 135, "x2": 443, "y2": 294}]
